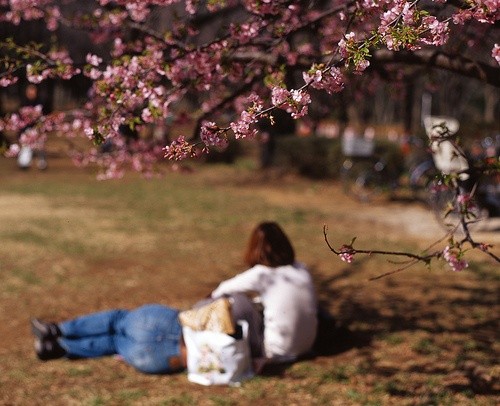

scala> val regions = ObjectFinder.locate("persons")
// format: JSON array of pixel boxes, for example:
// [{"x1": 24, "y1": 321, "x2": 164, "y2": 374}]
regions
[
  {"x1": 30, "y1": 292, "x2": 264, "y2": 375},
  {"x1": 212, "y1": 222, "x2": 319, "y2": 366}
]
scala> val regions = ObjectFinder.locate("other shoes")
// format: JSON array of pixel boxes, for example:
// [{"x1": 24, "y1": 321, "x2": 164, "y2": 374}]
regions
[
  {"x1": 34, "y1": 336, "x2": 57, "y2": 360},
  {"x1": 32, "y1": 319, "x2": 55, "y2": 339}
]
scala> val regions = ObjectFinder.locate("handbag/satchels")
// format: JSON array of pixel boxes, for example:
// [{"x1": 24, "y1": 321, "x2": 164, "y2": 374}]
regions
[{"x1": 182, "y1": 319, "x2": 254, "y2": 385}]
